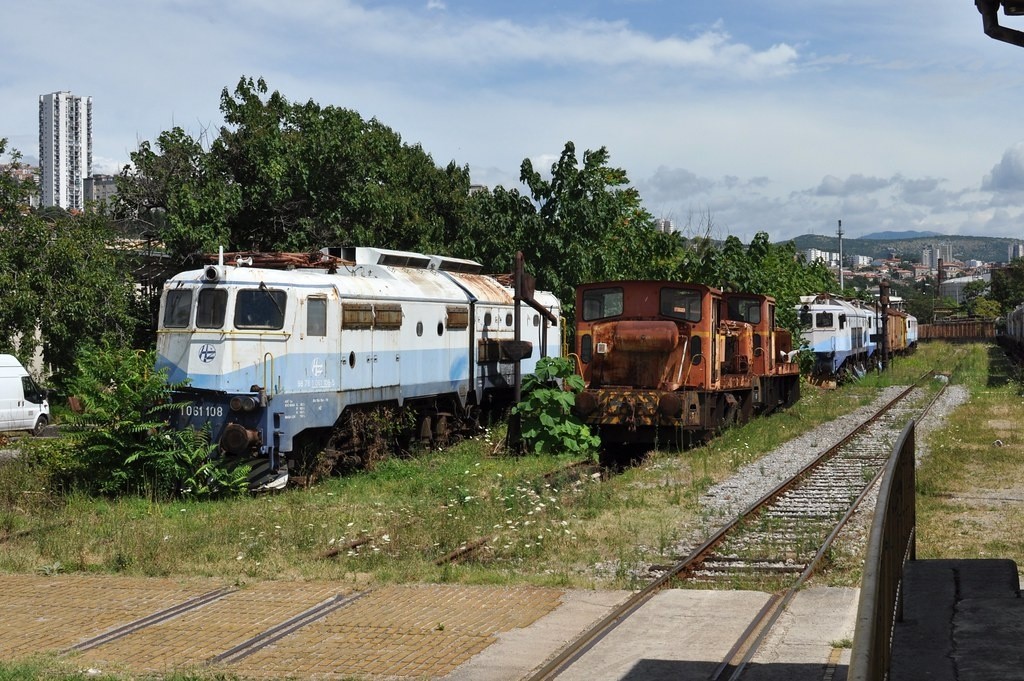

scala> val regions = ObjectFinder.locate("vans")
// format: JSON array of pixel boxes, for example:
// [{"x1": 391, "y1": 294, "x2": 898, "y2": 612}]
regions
[{"x1": 1, "y1": 352, "x2": 49, "y2": 436}]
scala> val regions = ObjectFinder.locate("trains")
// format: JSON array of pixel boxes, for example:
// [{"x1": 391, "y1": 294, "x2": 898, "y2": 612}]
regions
[
  {"x1": 910, "y1": 302, "x2": 1024, "y2": 357},
  {"x1": 793, "y1": 290, "x2": 919, "y2": 389},
  {"x1": 156, "y1": 243, "x2": 567, "y2": 498},
  {"x1": 569, "y1": 278, "x2": 800, "y2": 477}
]
